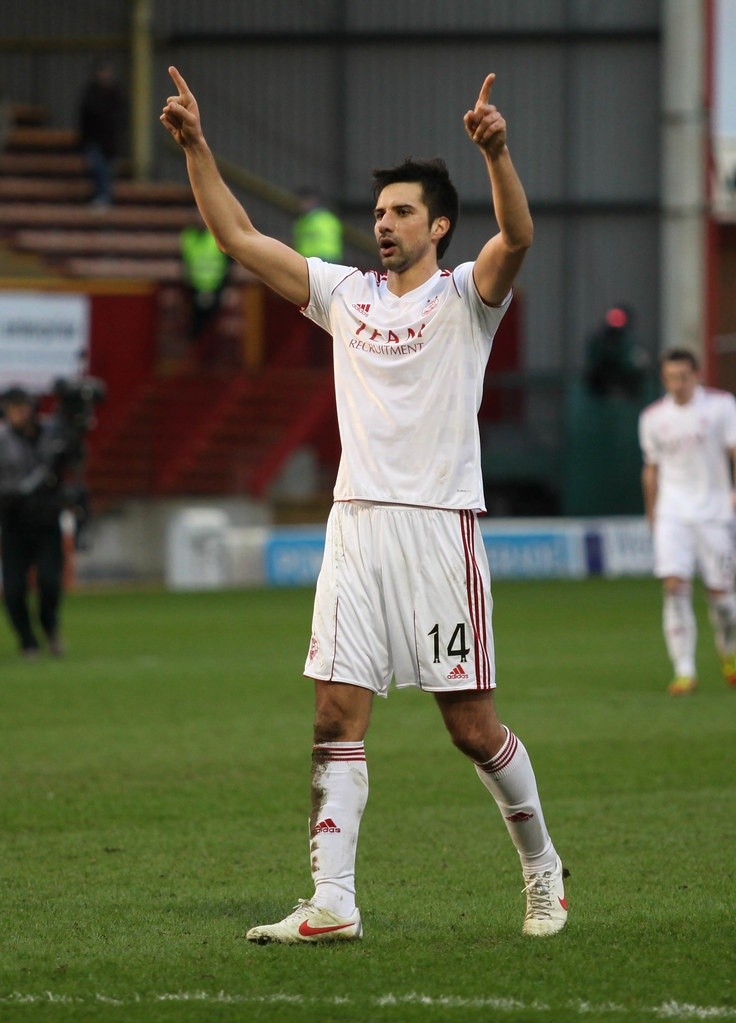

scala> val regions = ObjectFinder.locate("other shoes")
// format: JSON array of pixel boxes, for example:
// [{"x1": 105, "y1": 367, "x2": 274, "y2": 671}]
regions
[
  {"x1": 48, "y1": 637, "x2": 62, "y2": 656},
  {"x1": 22, "y1": 644, "x2": 37, "y2": 659},
  {"x1": 667, "y1": 679, "x2": 698, "y2": 695},
  {"x1": 725, "y1": 672, "x2": 736, "y2": 685}
]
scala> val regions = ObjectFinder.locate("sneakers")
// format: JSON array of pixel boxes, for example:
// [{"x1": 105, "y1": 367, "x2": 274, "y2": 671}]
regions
[
  {"x1": 247, "y1": 898, "x2": 363, "y2": 943},
  {"x1": 522, "y1": 854, "x2": 568, "y2": 936}
]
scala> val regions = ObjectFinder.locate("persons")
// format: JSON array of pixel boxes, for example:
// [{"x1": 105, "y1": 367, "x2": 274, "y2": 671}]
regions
[
  {"x1": 177, "y1": 202, "x2": 241, "y2": 366},
  {"x1": 636, "y1": 346, "x2": 736, "y2": 695},
  {"x1": 288, "y1": 185, "x2": 345, "y2": 365},
  {"x1": 158, "y1": 65, "x2": 575, "y2": 940},
  {"x1": 0, "y1": 384, "x2": 86, "y2": 658}
]
